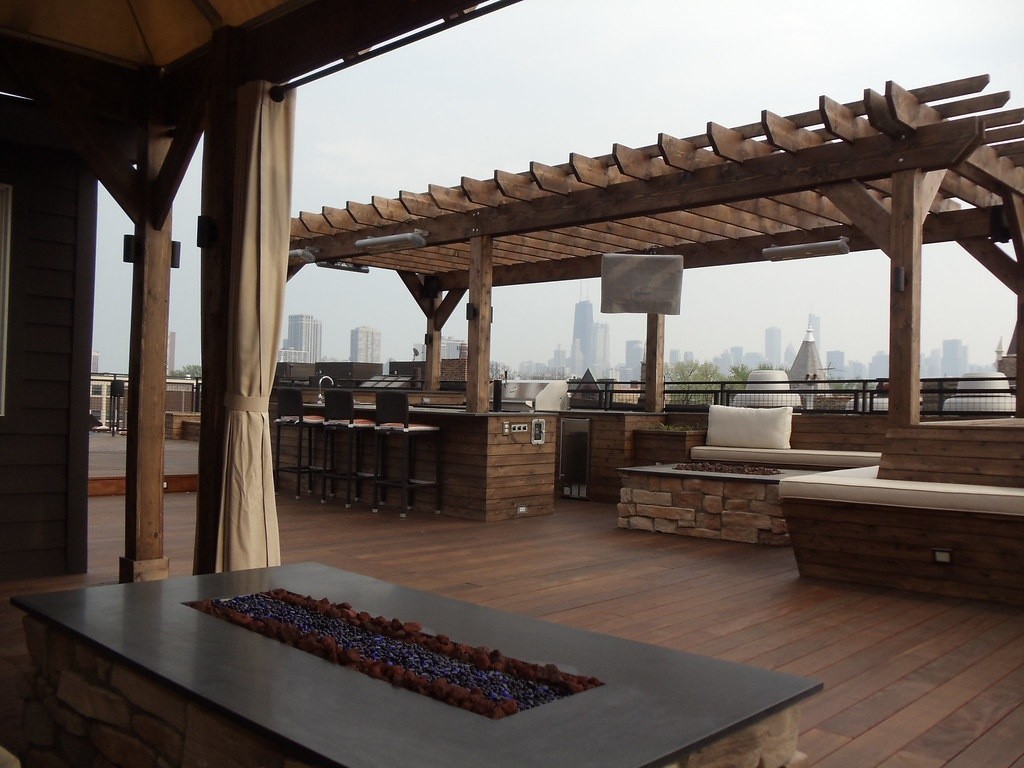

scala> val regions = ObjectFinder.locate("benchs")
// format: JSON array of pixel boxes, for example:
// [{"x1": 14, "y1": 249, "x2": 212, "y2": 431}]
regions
[{"x1": 690, "y1": 445, "x2": 1024, "y2": 609}]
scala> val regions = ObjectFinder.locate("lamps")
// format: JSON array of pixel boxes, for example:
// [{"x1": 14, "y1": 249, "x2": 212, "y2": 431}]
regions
[
  {"x1": 197, "y1": 216, "x2": 216, "y2": 247},
  {"x1": 316, "y1": 257, "x2": 369, "y2": 273},
  {"x1": 762, "y1": 236, "x2": 850, "y2": 261},
  {"x1": 124, "y1": 235, "x2": 136, "y2": 263},
  {"x1": 467, "y1": 303, "x2": 476, "y2": 321},
  {"x1": 171, "y1": 241, "x2": 180, "y2": 268},
  {"x1": 932, "y1": 547, "x2": 953, "y2": 566},
  {"x1": 893, "y1": 266, "x2": 908, "y2": 293},
  {"x1": 491, "y1": 307, "x2": 493, "y2": 323},
  {"x1": 288, "y1": 245, "x2": 321, "y2": 267},
  {"x1": 425, "y1": 334, "x2": 432, "y2": 345},
  {"x1": 354, "y1": 228, "x2": 429, "y2": 255}
]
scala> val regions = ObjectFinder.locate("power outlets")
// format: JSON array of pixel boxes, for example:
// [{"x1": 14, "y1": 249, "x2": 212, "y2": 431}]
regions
[{"x1": 503, "y1": 420, "x2": 510, "y2": 436}]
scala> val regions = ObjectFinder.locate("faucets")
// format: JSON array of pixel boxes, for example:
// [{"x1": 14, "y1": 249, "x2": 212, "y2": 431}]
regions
[{"x1": 317, "y1": 376, "x2": 334, "y2": 405}]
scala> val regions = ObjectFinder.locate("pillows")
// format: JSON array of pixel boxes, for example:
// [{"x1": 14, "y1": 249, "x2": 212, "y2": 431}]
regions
[{"x1": 706, "y1": 404, "x2": 794, "y2": 450}]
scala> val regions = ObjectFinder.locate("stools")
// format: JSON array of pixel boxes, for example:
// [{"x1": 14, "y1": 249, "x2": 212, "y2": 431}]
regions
[
  {"x1": 321, "y1": 390, "x2": 385, "y2": 509},
  {"x1": 272, "y1": 388, "x2": 336, "y2": 500},
  {"x1": 372, "y1": 391, "x2": 442, "y2": 517}
]
